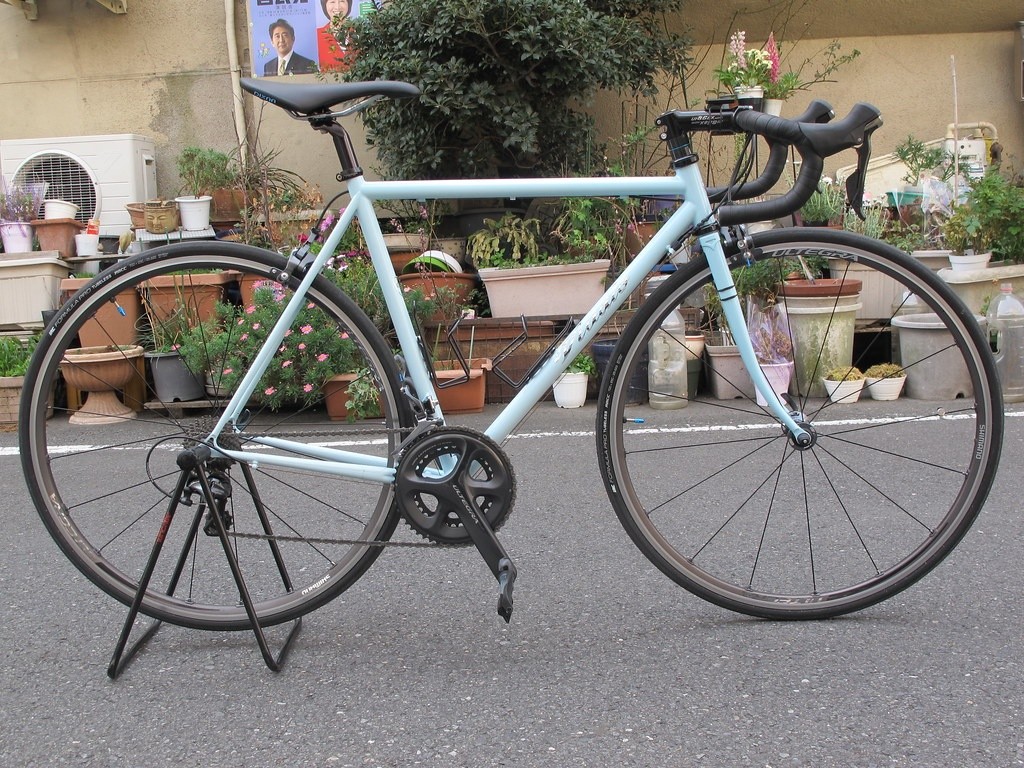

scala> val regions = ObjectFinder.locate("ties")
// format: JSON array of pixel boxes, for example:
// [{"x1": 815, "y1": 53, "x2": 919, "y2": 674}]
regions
[{"x1": 278, "y1": 60, "x2": 286, "y2": 76}]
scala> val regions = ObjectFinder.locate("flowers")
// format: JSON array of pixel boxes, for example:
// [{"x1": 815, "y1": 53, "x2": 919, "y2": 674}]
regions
[
  {"x1": 725, "y1": 27, "x2": 781, "y2": 86},
  {"x1": 171, "y1": 207, "x2": 437, "y2": 421}
]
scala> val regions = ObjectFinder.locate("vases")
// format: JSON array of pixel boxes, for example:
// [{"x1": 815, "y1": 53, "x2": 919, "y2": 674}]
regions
[
  {"x1": 828, "y1": 193, "x2": 907, "y2": 319},
  {"x1": 891, "y1": 313, "x2": 987, "y2": 400},
  {"x1": 763, "y1": 97, "x2": 783, "y2": 116},
  {"x1": 398, "y1": 272, "x2": 476, "y2": 320},
  {"x1": 430, "y1": 233, "x2": 468, "y2": 264},
  {"x1": 421, "y1": 317, "x2": 555, "y2": 403},
  {"x1": 31, "y1": 218, "x2": 85, "y2": 257},
  {"x1": 125, "y1": 201, "x2": 145, "y2": 226},
  {"x1": 74, "y1": 234, "x2": 99, "y2": 256},
  {"x1": 774, "y1": 278, "x2": 862, "y2": 398}
]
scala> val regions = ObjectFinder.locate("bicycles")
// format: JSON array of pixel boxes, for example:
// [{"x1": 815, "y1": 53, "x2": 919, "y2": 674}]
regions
[{"x1": 18, "y1": 75, "x2": 1008, "y2": 680}]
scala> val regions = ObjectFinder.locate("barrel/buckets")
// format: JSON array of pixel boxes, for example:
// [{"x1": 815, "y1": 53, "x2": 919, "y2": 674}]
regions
[
  {"x1": 776, "y1": 293, "x2": 861, "y2": 396},
  {"x1": 776, "y1": 279, "x2": 863, "y2": 296},
  {"x1": 890, "y1": 313, "x2": 985, "y2": 401}
]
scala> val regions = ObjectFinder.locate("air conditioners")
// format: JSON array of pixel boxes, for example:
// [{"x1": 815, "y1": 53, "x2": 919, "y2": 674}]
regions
[{"x1": 0, "y1": 134, "x2": 156, "y2": 238}]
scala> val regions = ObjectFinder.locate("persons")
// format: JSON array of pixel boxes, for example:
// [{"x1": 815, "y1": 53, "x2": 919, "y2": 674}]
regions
[
  {"x1": 317, "y1": 0, "x2": 365, "y2": 73},
  {"x1": 264, "y1": 19, "x2": 318, "y2": 77}
]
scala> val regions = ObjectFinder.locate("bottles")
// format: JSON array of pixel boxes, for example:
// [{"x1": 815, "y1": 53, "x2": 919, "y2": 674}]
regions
[
  {"x1": 648, "y1": 303, "x2": 688, "y2": 410},
  {"x1": 986, "y1": 283, "x2": 1024, "y2": 404}
]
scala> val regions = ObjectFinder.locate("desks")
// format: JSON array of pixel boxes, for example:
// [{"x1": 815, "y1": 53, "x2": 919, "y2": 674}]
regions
[{"x1": 903, "y1": 224, "x2": 954, "y2": 271}]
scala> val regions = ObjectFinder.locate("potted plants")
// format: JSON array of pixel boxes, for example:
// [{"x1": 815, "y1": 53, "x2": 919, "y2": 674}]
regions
[
  {"x1": 59, "y1": 345, "x2": 143, "y2": 426},
  {"x1": 592, "y1": 318, "x2": 649, "y2": 407},
  {"x1": 626, "y1": 200, "x2": 664, "y2": 264},
  {"x1": 0, "y1": 331, "x2": 53, "y2": 424},
  {"x1": 60, "y1": 271, "x2": 141, "y2": 346},
  {"x1": 553, "y1": 352, "x2": 596, "y2": 409},
  {"x1": 823, "y1": 366, "x2": 865, "y2": 404},
  {"x1": 941, "y1": 201, "x2": 993, "y2": 271},
  {"x1": 175, "y1": 146, "x2": 234, "y2": 230},
  {"x1": 139, "y1": 268, "x2": 235, "y2": 346},
  {"x1": 320, "y1": 323, "x2": 491, "y2": 422},
  {"x1": 884, "y1": 134, "x2": 944, "y2": 207},
  {"x1": 236, "y1": 227, "x2": 292, "y2": 312},
  {"x1": 936, "y1": 165, "x2": 1024, "y2": 315},
  {"x1": 685, "y1": 325, "x2": 706, "y2": 360},
  {"x1": 749, "y1": 309, "x2": 794, "y2": 407},
  {"x1": 43, "y1": 195, "x2": 80, "y2": 220},
  {"x1": 205, "y1": 147, "x2": 306, "y2": 220},
  {"x1": 250, "y1": 182, "x2": 339, "y2": 245},
  {"x1": 477, "y1": 248, "x2": 612, "y2": 318},
  {"x1": 144, "y1": 309, "x2": 207, "y2": 403},
  {"x1": 785, "y1": 160, "x2": 829, "y2": 227},
  {"x1": 0, "y1": 171, "x2": 45, "y2": 253},
  {"x1": 207, "y1": 299, "x2": 234, "y2": 395},
  {"x1": 703, "y1": 283, "x2": 755, "y2": 401},
  {"x1": 866, "y1": 362, "x2": 907, "y2": 400}
]
